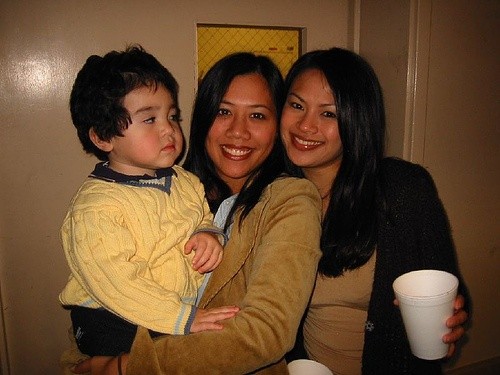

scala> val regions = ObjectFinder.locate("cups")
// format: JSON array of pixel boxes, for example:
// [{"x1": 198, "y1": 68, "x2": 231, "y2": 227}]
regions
[
  {"x1": 393, "y1": 269, "x2": 459, "y2": 360},
  {"x1": 287, "y1": 359, "x2": 333, "y2": 375}
]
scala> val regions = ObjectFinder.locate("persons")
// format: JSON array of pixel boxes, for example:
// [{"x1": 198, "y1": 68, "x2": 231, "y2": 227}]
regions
[
  {"x1": 58, "y1": 43, "x2": 240, "y2": 355},
  {"x1": 276, "y1": 46, "x2": 473, "y2": 375},
  {"x1": 58, "y1": 51, "x2": 322, "y2": 375}
]
